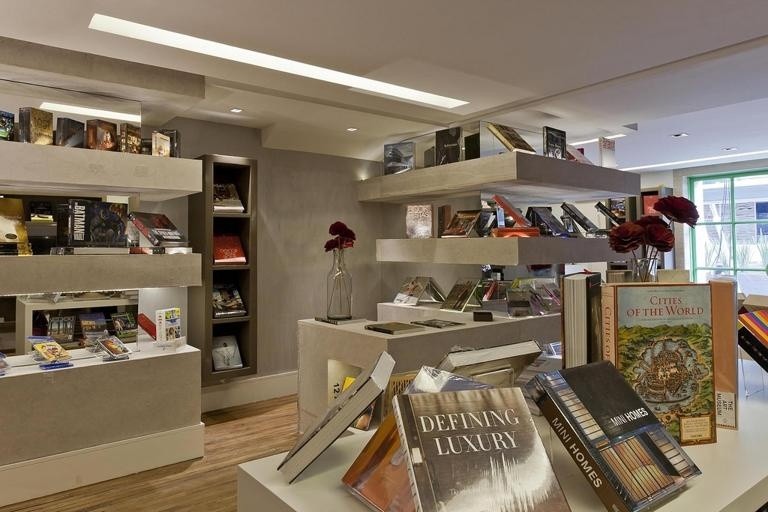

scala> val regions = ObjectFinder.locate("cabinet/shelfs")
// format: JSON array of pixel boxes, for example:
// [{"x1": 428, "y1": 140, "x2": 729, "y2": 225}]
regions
[
  {"x1": 0, "y1": 138, "x2": 204, "y2": 507},
  {"x1": 189, "y1": 154, "x2": 258, "y2": 388},
  {"x1": 357, "y1": 150, "x2": 641, "y2": 323}
]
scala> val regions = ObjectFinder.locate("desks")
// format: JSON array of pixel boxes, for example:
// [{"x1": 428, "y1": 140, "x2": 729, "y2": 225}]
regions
[{"x1": 237, "y1": 359, "x2": 768, "y2": 509}]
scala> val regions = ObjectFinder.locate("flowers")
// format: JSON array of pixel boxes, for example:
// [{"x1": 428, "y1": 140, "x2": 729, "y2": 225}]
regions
[
  {"x1": 606, "y1": 195, "x2": 700, "y2": 278},
  {"x1": 322, "y1": 221, "x2": 358, "y2": 315}
]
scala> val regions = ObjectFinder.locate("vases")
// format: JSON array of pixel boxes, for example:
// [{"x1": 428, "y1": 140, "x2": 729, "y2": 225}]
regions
[
  {"x1": 327, "y1": 248, "x2": 354, "y2": 321},
  {"x1": 629, "y1": 255, "x2": 660, "y2": 284}
]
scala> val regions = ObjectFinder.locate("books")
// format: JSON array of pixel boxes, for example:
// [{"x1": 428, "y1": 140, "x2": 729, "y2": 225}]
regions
[
  {"x1": 213, "y1": 183, "x2": 244, "y2": 213},
  {"x1": 212, "y1": 283, "x2": 247, "y2": 318},
  {"x1": 213, "y1": 234, "x2": 246, "y2": 265},
  {"x1": 212, "y1": 335, "x2": 243, "y2": 371}
]
